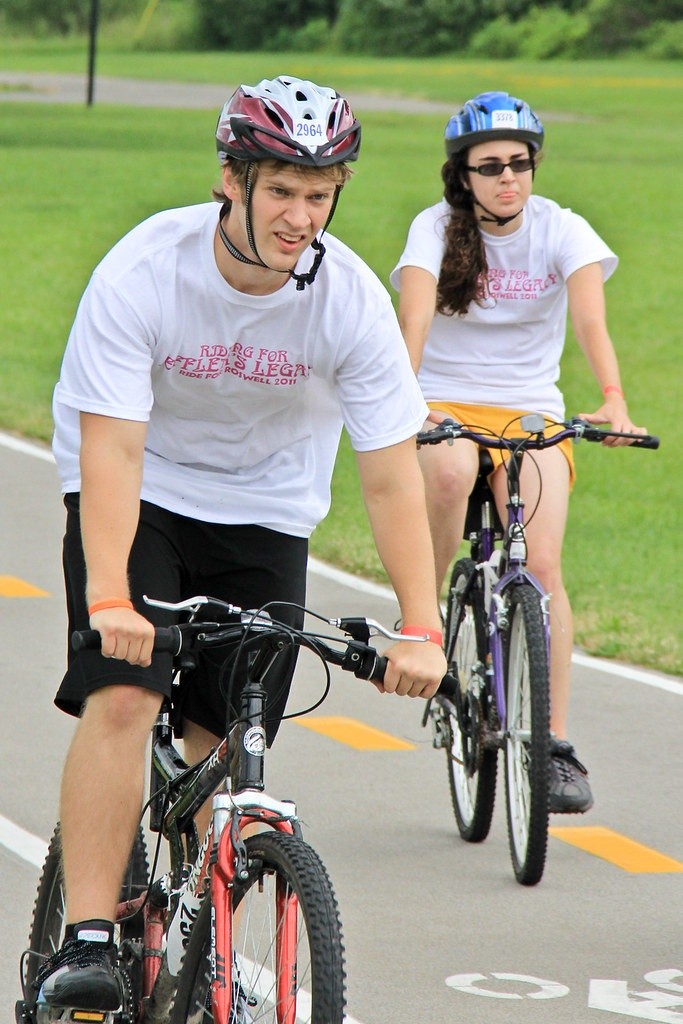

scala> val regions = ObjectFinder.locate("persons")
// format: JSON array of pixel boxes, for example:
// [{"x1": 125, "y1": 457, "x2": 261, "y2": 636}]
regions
[
  {"x1": 34, "y1": 75, "x2": 446, "y2": 1017},
  {"x1": 390, "y1": 92, "x2": 650, "y2": 815}
]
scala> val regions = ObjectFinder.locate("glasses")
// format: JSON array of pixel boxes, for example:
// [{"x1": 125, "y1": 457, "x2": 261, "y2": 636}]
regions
[{"x1": 462, "y1": 158, "x2": 535, "y2": 177}]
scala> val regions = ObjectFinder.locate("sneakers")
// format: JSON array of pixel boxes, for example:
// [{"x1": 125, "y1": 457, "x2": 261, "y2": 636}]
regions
[
  {"x1": 202, "y1": 970, "x2": 247, "y2": 1024},
  {"x1": 40, "y1": 919, "x2": 122, "y2": 1012},
  {"x1": 546, "y1": 738, "x2": 594, "y2": 813}
]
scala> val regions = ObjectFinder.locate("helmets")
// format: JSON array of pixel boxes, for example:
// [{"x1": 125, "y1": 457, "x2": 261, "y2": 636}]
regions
[
  {"x1": 444, "y1": 91, "x2": 545, "y2": 159},
  {"x1": 214, "y1": 75, "x2": 361, "y2": 167}
]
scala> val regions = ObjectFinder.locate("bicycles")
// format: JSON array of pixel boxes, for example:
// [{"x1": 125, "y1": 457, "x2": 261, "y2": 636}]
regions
[
  {"x1": 12, "y1": 593, "x2": 458, "y2": 1024},
  {"x1": 415, "y1": 414, "x2": 660, "y2": 885}
]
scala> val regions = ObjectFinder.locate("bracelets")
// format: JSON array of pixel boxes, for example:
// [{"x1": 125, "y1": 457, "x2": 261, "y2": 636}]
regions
[
  {"x1": 601, "y1": 386, "x2": 623, "y2": 397},
  {"x1": 400, "y1": 626, "x2": 444, "y2": 647},
  {"x1": 87, "y1": 598, "x2": 134, "y2": 614}
]
